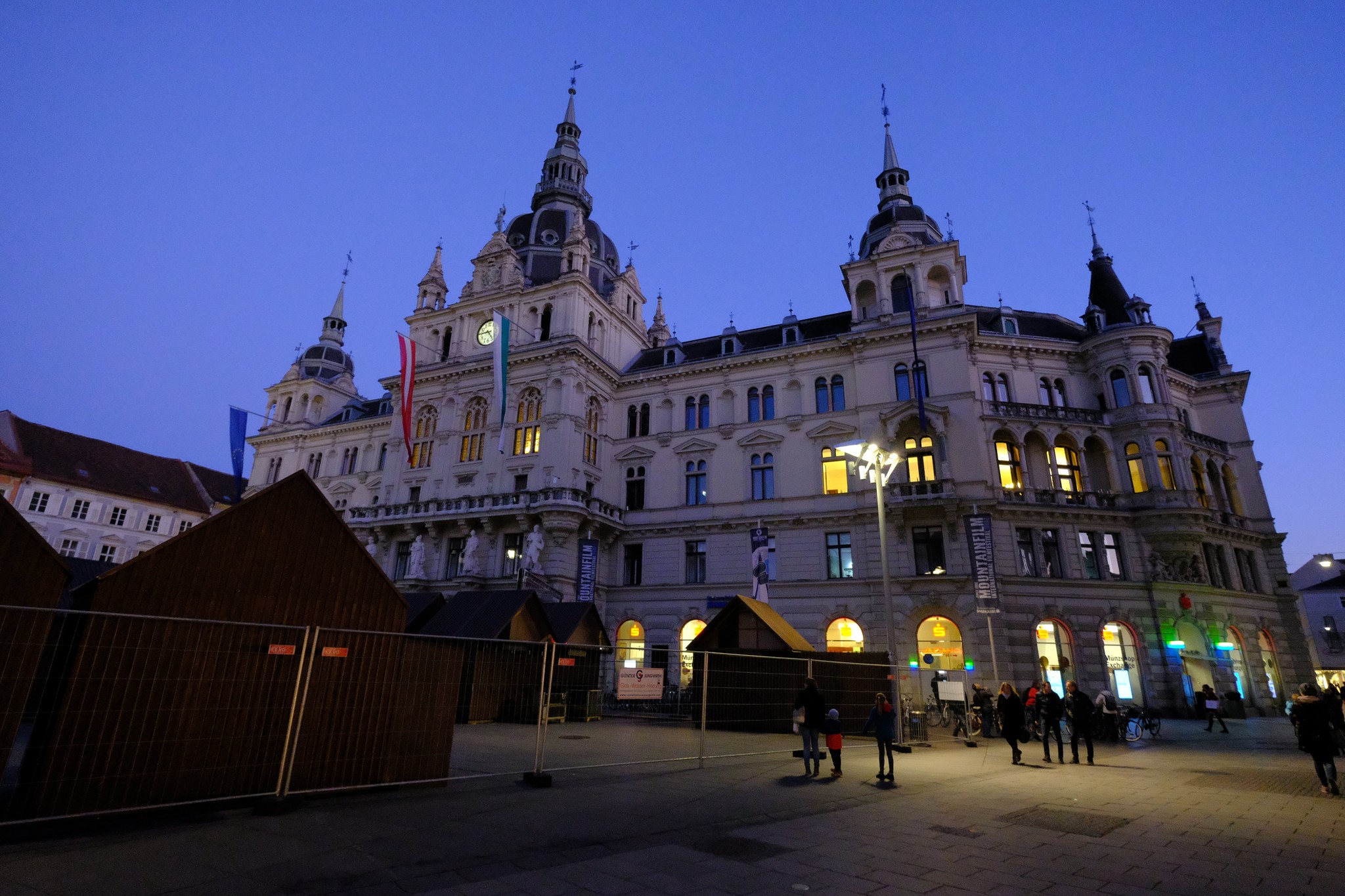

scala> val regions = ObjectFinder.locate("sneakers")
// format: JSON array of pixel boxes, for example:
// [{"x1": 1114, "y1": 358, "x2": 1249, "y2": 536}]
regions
[
  {"x1": 1069, "y1": 757, "x2": 1080, "y2": 764},
  {"x1": 1321, "y1": 786, "x2": 1329, "y2": 793},
  {"x1": 814, "y1": 768, "x2": 819, "y2": 775},
  {"x1": 1058, "y1": 757, "x2": 1064, "y2": 763},
  {"x1": 1087, "y1": 760, "x2": 1094, "y2": 766},
  {"x1": 1328, "y1": 778, "x2": 1339, "y2": 792},
  {"x1": 831, "y1": 767, "x2": 843, "y2": 776},
  {"x1": 876, "y1": 773, "x2": 885, "y2": 779},
  {"x1": 885, "y1": 774, "x2": 894, "y2": 780},
  {"x1": 805, "y1": 767, "x2": 811, "y2": 775},
  {"x1": 1042, "y1": 757, "x2": 1052, "y2": 762},
  {"x1": 831, "y1": 768, "x2": 836, "y2": 773}
]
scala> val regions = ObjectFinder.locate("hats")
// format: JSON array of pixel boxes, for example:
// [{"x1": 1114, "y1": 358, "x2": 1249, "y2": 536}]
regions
[{"x1": 828, "y1": 709, "x2": 839, "y2": 720}]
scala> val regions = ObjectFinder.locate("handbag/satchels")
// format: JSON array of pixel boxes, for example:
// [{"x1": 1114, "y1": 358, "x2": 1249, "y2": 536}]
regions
[
  {"x1": 1329, "y1": 712, "x2": 1345, "y2": 749},
  {"x1": 1019, "y1": 725, "x2": 1030, "y2": 743},
  {"x1": 793, "y1": 706, "x2": 805, "y2": 733}
]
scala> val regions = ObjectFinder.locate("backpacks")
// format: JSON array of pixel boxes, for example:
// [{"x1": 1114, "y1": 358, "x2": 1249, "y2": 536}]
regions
[
  {"x1": 1101, "y1": 693, "x2": 1115, "y2": 710},
  {"x1": 1022, "y1": 687, "x2": 1033, "y2": 701}
]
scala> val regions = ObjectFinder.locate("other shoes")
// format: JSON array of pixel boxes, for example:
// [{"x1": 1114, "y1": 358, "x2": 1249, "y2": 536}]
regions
[
  {"x1": 1298, "y1": 745, "x2": 1306, "y2": 751},
  {"x1": 1219, "y1": 729, "x2": 1229, "y2": 733},
  {"x1": 1341, "y1": 748, "x2": 1345, "y2": 756},
  {"x1": 1110, "y1": 738, "x2": 1118, "y2": 742},
  {"x1": 1204, "y1": 727, "x2": 1212, "y2": 732},
  {"x1": 986, "y1": 734, "x2": 994, "y2": 738},
  {"x1": 982, "y1": 734, "x2": 987, "y2": 737},
  {"x1": 1333, "y1": 750, "x2": 1340, "y2": 757},
  {"x1": 1100, "y1": 735, "x2": 1108, "y2": 740}
]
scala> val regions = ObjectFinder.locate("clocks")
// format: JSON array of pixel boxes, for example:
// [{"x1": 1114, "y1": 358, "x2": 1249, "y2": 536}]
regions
[{"x1": 477, "y1": 319, "x2": 501, "y2": 346}]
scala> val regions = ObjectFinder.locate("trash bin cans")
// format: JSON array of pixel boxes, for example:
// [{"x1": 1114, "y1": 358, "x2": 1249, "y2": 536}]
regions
[{"x1": 907, "y1": 710, "x2": 931, "y2": 748}]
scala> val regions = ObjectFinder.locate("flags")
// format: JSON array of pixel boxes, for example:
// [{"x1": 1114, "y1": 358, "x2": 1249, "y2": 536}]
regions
[
  {"x1": 396, "y1": 334, "x2": 416, "y2": 463},
  {"x1": 490, "y1": 310, "x2": 511, "y2": 454},
  {"x1": 228, "y1": 406, "x2": 248, "y2": 501}
]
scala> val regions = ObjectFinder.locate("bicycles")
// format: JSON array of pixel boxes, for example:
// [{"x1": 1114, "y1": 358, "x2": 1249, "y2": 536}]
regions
[
  {"x1": 921, "y1": 695, "x2": 942, "y2": 726},
  {"x1": 958, "y1": 697, "x2": 1163, "y2": 742},
  {"x1": 897, "y1": 693, "x2": 917, "y2": 729},
  {"x1": 941, "y1": 700, "x2": 952, "y2": 727}
]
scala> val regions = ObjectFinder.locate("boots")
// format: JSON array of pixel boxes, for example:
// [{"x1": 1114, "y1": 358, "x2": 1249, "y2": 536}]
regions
[
  {"x1": 1016, "y1": 748, "x2": 1021, "y2": 761},
  {"x1": 1012, "y1": 749, "x2": 1018, "y2": 763}
]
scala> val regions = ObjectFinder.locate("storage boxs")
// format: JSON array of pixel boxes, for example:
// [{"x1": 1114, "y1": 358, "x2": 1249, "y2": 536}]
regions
[{"x1": 524, "y1": 685, "x2": 603, "y2": 725}]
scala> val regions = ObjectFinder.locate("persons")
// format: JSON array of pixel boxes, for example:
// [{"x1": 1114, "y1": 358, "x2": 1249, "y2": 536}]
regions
[
  {"x1": 819, "y1": 707, "x2": 845, "y2": 778},
  {"x1": 794, "y1": 677, "x2": 825, "y2": 776},
  {"x1": 950, "y1": 691, "x2": 970, "y2": 740},
  {"x1": 972, "y1": 683, "x2": 996, "y2": 737},
  {"x1": 930, "y1": 670, "x2": 947, "y2": 713},
  {"x1": 1285, "y1": 678, "x2": 1344, "y2": 793},
  {"x1": 461, "y1": 529, "x2": 480, "y2": 575},
  {"x1": 410, "y1": 535, "x2": 425, "y2": 574},
  {"x1": 523, "y1": 524, "x2": 545, "y2": 571},
  {"x1": 1095, "y1": 689, "x2": 1120, "y2": 745},
  {"x1": 866, "y1": 692, "x2": 897, "y2": 781},
  {"x1": 1063, "y1": 680, "x2": 1096, "y2": 765},
  {"x1": 365, "y1": 537, "x2": 376, "y2": 557},
  {"x1": 996, "y1": 682, "x2": 1026, "y2": 765},
  {"x1": 494, "y1": 203, "x2": 507, "y2": 232},
  {"x1": 1025, "y1": 680, "x2": 1042, "y2": 739},
  {"x1": 1201, "y1": 684, "x2": 1229, "y2": 733},
  {"x1": 1031, "y1": 681, "x2": 1067, "y2": 763}
]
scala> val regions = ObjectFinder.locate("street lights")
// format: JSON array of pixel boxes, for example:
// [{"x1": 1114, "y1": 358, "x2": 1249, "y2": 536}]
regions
[{"x1": 834, "y1": 440, "x2": 911, "y2": 749}]
